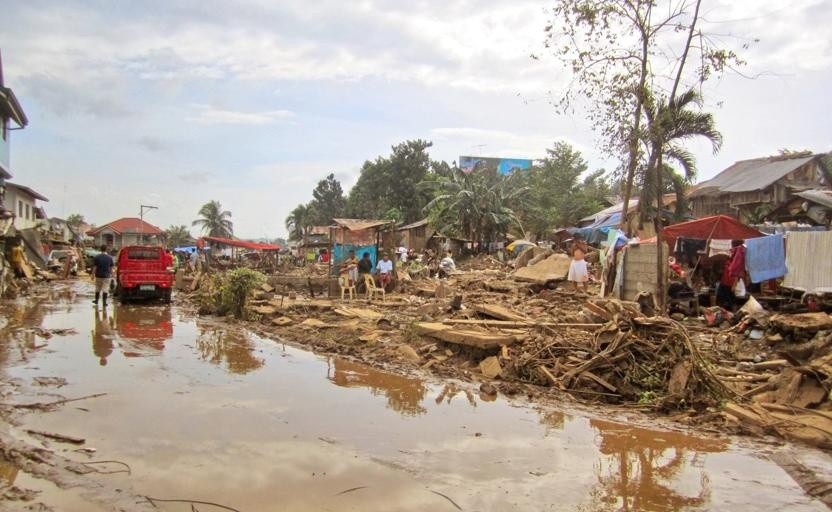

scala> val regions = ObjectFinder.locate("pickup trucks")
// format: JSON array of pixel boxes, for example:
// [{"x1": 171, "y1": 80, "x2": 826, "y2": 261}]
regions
[
  {"x1": 115, "y1": 244, "x2": 174, "y2": 304},
  {"x1": 113, "y1": 305, "x2": 173, "y2": 359}
]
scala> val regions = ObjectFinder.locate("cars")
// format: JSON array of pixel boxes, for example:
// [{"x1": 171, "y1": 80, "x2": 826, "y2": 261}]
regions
[{"x1": 45, "y1": 249, "x2": 77, "y2": 275}]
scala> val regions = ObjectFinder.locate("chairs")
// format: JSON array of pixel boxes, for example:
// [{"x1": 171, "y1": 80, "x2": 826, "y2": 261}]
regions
[
  {"x1": 338, "y1": 273, "x2": 357, "y2": 300},
  {"x1": 364, "y1": 273, "x2": 385, "y2": 301}
]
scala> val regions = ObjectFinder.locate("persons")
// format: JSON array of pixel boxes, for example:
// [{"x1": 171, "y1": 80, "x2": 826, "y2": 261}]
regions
[
  {"x1": 345, "y1": 250, "x2": 360, "y2": 283},
  {"x1": 394, "y1": 244, "x2": 408, "y2": 263},
  {"x1": 727, "y1": 239, "x2": 747, "y2": 281},
  {"x1": 357, "y1": 253, "x2": 372, "y2": 294},
  {"x1": 567, "y1": 232, "x2": 589, "y2": 292},
  {"x1": 189, "y1": 245, "x2": 205, "y2": 291},
  {"x1": 376, "y1": 252, "x2": 392, "y2": 292},
  {"x1": 91, "y1": 245, "x2": 114, "y2": 306},
  {"x1": 171, "y1": 251, "x2": 178, "y2": 276},
  {"x1": 319, "y1": 249, "x2": 328, "y2": 263},
  {"x1": 10, "y1": 240, "x2": 29, "y2": 281}
]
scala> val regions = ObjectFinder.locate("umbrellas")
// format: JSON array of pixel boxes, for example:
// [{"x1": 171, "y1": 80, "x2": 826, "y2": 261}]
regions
[{"x1": 658, "y1": 214, "x2": 770, "y2": 241}]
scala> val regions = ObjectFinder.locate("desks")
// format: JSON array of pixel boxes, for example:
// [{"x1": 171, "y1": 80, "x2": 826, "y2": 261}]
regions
[{"x1": 757, "y1": 295, "x2": 786, "y2": 310}]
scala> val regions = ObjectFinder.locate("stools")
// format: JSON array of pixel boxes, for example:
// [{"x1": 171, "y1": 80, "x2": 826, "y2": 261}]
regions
[{"x1": 669, "y1": 296, "x2": 701, "y2": 316}]
